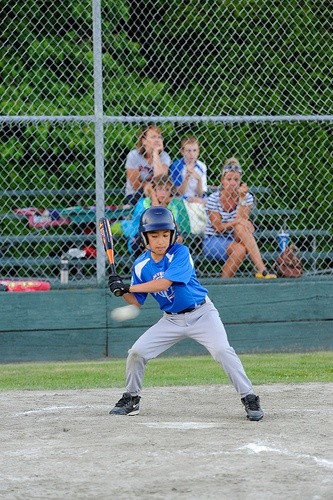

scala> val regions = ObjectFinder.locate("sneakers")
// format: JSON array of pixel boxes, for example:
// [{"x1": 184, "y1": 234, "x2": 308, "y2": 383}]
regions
[
  {"x1": 110, "y1": 393, "x2": 140, "y2": 417},
  {"x1": 241, "y1": 394, "x2": 263, "y2": 421}
]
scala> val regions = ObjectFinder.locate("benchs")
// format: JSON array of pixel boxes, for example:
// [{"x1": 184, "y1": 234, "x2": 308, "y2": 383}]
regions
[
  {"x1": 0, "y1": 187, "x2": 271, "y2": 205},
  {"x1": 0, "y1": 251, "x2": 333, "y2": 280},
  {"x1": 0, "y1": 206, "x2": 302, "y2": 232},
  {"x1": 0, "y1": 229, "x2": 331, "y2": 258}
]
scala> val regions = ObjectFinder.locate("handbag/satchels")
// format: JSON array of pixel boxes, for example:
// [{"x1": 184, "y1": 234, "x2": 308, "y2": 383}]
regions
[{"x1": 275, "y1": 245, "x2": 302, "y2": 278}]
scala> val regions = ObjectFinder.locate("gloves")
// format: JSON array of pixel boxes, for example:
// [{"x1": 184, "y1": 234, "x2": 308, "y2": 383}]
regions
[{"x1": 108, "y1": 273, "x2": 130, "y2": 297}]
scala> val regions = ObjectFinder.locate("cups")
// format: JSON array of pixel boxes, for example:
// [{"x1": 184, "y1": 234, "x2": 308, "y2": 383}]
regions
[{"x1": 278, "y1": 230, "x2": 290, "y2": 254}]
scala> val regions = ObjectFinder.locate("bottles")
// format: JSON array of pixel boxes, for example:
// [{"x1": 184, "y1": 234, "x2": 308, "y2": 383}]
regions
[{"x1": 60, "y1": 250, "x2": 70, "y2": 285}]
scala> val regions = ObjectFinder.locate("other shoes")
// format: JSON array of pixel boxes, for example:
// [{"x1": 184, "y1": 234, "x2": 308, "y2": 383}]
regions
[{"x1": 255, "y1": 270, "x2": 277, "y2": 280}]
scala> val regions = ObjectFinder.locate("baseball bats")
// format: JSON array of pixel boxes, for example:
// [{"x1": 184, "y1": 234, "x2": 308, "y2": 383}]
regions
[{"x1": 98, "y1": 217, "x2": 120, "y2": 297}]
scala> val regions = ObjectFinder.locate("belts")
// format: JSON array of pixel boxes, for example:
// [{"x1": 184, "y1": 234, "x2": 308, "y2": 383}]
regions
[{"x1": 165, "y1": 300, "x2": 205, "y2": 316}]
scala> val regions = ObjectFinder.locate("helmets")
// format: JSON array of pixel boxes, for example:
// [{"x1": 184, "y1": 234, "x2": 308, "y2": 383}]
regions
[{"x1": 137, "y1": 205, "x2": 178, "y2": 245}]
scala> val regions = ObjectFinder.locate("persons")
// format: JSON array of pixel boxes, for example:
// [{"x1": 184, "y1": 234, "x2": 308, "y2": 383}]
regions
[
  {"x1": 121, "y1": 173, "x2": 191, "y2": 261},
  {"x1": 124, "y1": 124, "x2": 174, "y2": 208},
  {"x1": 203, "y1": 155, "x2": 278, "y2": 278},
  {"x1": 167, "y1": 133, "x2": 208, "y2": 235},
  {"x1": 109, "y1": 207, "x2": 264, "y2": 421}
]
